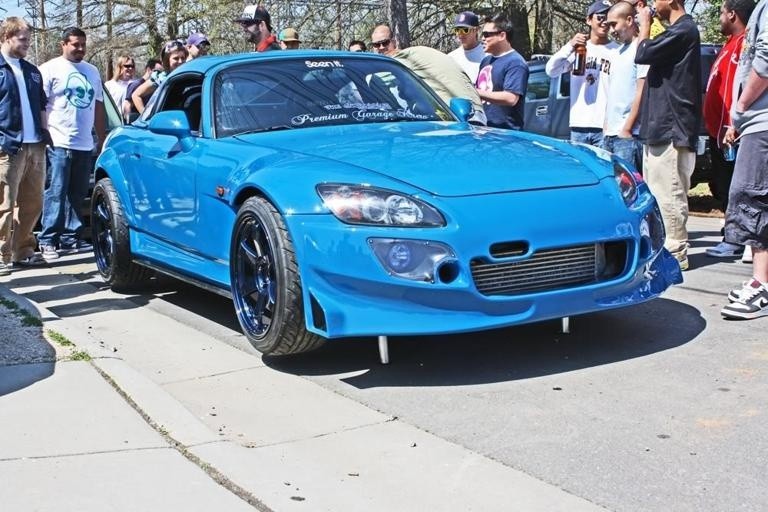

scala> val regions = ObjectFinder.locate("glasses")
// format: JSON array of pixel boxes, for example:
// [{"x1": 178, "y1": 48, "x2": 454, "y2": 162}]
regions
[
  {"x1": 152, "y1": 69, "x2": 161, "y2": 74},
  {"x1": 354, "y1": 49, "x2": 363, "y2": 52},
  {"x1": 370, "y1": 36, "x2": 394, "y2": 48},
  {"x1": 454, "y1": 27, "x2": 474, "y2": 35},
  {"x1": 167, "y1": 41, "x2": 183, "y2": 48},
  {"x1": 480, "y1": 30, "x2": 502, "y2": 38},
  {"x1": 592, "y1": 14, "x2": 608, "y2": 22},
  {"x1": 121, "y1": 64, "x2": 135, "y2": 69}
]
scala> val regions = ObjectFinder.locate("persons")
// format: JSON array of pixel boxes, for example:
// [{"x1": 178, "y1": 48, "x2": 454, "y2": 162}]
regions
[
  {"x1": 0, "y1": 16, "x2": 55, "y2": 277},
  {"x1": 719, "y1": 1, "x2": 767, "y2": 320}
]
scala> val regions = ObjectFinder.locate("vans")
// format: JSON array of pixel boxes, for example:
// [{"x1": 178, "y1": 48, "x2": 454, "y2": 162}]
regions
[{"x1": 517, "y1": 44, "x2": 737, "y2": 205}]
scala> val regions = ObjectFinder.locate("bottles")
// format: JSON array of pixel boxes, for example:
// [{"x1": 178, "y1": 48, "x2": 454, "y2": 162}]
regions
[{"x1": 572, "y1": 27, "x2": 588, "y2": 78}]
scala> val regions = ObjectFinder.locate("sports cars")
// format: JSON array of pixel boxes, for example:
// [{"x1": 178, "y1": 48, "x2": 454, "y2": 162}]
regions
[{"x1": 86, "y1": 48, "x2": 688, "y2": 360}]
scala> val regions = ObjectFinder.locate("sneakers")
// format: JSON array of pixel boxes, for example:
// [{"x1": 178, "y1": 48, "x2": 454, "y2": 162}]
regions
[
  {"x1": 670, "y1": 247, "x2": 691, "y2": 272},
  {"x1": 703, "y1": 238, "x2": 746, "y2": 260},
  {"x1": 13, "y1": 255, "x2": 48, "y2": 266},
  {"x1": 0, "y1": 261, "x2": 11, "y2": 276},
  {"x1": 38, "y1": 243, "x2": 60, "y2": 259},
  {"x1": 58, "y1": 235, "x2": 94, "y2": 253},
  {"x1": 721, "y1": 275, "x2": 768, "y2": 322}
]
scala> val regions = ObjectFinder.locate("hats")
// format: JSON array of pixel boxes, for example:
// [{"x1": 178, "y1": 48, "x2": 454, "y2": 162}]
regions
[
  {"x1": 186, "y1": 31, "x2": 211, "y2": 48},
  {"x1": 233, "y1": 4, "x2": 271, "y2": 25},
  {"x1": 586, "y1": 0, "x2": 614, "y2": 18},
  {"x1": 452, "y1": 10, "x2": 482, "y2": 28},
  {"x1": 278, "y1": 27, "x2": 303, "y2": 44}
]
